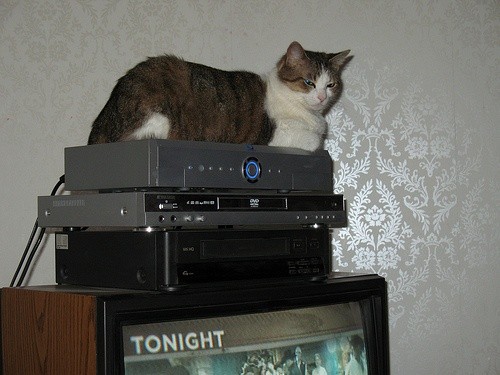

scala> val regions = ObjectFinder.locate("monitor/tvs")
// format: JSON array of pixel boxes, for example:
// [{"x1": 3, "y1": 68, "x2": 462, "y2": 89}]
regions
[{"x1": 0, "y1": 271, "x2": 392, "y2": 374}]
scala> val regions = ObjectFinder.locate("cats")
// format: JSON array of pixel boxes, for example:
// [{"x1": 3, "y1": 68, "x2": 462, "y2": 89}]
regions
[{"x1": 87, "y1": 41, "x2": 352, "y2": 151}]
